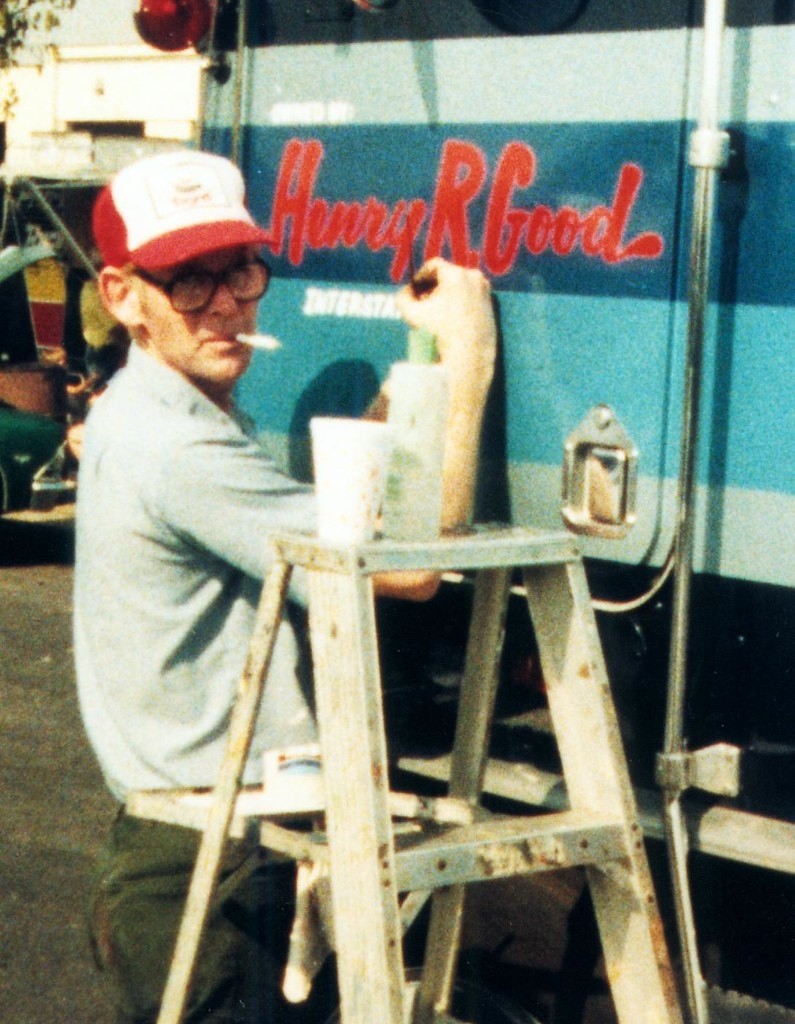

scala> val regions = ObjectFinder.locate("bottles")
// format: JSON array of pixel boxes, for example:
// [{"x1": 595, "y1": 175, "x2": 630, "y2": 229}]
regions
[{"x1": 386, "y1": 329, "x2": 449, "y2": 538}]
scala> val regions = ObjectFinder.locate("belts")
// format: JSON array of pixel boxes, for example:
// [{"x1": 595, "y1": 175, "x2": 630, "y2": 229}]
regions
[{"x1": 125, "y1": 777, "x2": 327, "y2": 861}]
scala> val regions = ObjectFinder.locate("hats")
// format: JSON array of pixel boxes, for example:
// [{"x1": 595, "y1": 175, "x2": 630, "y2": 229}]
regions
[{"x1": 93, "y1": 150, "x2": 277, "y2": 273}]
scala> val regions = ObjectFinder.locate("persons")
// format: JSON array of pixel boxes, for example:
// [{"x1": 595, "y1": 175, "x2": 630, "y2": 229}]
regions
[{"x1": 73, "y1": 150, "x2": 496, "y2": 1024}]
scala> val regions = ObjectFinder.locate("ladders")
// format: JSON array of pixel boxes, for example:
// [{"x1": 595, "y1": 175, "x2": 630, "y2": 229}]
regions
[{"x1": 157, "y1": 533, "x2": 690, "y2": 1023}]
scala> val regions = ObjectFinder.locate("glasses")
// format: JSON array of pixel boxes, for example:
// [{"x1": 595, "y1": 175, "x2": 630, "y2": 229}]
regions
[{"x1": 127, "y1": 247, "x2": 271, "y2": 314}]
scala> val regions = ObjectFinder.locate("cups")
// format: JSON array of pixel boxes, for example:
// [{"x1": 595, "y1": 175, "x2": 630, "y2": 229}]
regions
[{"x1": 309, "y1": 414, "x2": 395, "y2": 544}]
